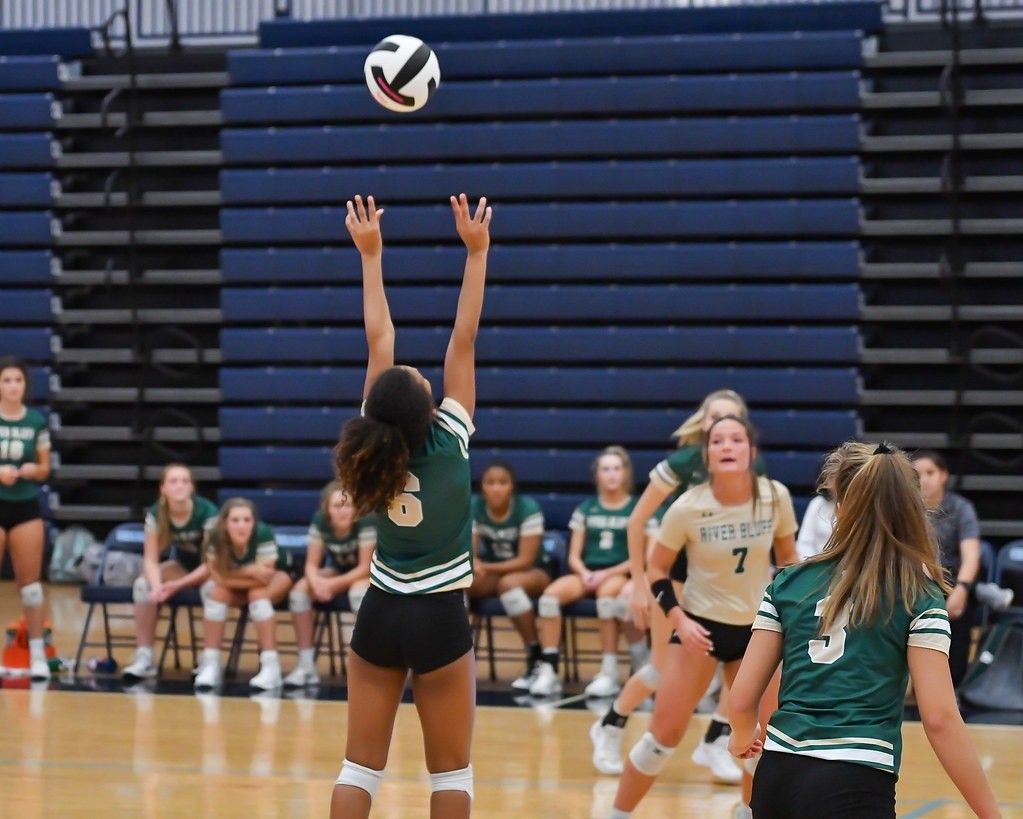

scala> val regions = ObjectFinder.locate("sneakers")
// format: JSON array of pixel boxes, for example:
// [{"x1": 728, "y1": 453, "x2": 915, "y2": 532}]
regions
[
  {"x1": 122, "y1": 678, "x2": 154, "y2": 699},
  {"x1": 585, "y1": 672, "x2": 619, "y2": 696},
  {"x1": 284, "y1": 661, "x2": 320, "y2": 686},
  {"x1": 284, "y1": 687, "x2": 318, "y2": 702},
  {"x1": 511, "y1": 667, "x2": 539, "y2": 690},
  {"x1": 530, "y1": 663, "x2": 562, "y2": 695},
  {"x1": 194, "y1": 688, "x2": 219, "y2": 702},
  {"x1": 692, "y1": 735, "x2": 743, "y2": 783},
  {"x1": 30, "y1": 652, "x2": 50, "y2": 679},
  {"x1": 192, "y1": 661, "x2": 221, "y2": 688},
  {"x1": 590, "y1": 716, "x2": 624, "y2": 774},
  {"x1": 122, "y1": 657, "x2": 157, "y2": 681},
  {"x1": 250, "y1": 689, "x2": 280, "y2": 705},
  {"x1": 249, "y1": 663, "x2": 282, "y2": 689}
]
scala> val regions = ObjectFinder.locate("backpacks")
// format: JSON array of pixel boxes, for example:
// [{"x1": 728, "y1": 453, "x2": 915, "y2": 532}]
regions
[{"x1": 50, "y1": 525, "x2": 97, "y2": 582}]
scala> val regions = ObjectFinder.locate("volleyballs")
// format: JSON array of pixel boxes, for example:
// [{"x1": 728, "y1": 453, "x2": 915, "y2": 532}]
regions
[{"x1": 363, "y1": 34, "x2": 441, "y2": 113}]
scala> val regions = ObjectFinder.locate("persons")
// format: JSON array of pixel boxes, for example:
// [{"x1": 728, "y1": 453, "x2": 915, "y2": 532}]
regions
[
  {"x1": 123, "y1": 464, "x2": 220, "y2": 679},
  {"x1": 913, "y1": 450, "x2": 981, "y2": 685},
  {"x1": 730, "y1": 438, "x2": 1002, "y2": 819},
  {"x1": 329, "y1": 194, "x2": 492, "y2": 819},
  {"x1": 464, "y1": 460, "x2": 561, "y2": 690},
  {"x1": 0, "y1": 354, "x2": 52, "y2": 680},
  {"x1": 590, "y1": 388, "x2": 744, "y2": 782},
  {"x1": 532, "y1": 447, "x2": 637, "y2": 697},
  {"x1": 283, "y1": 481, "x2": 378, "y2": 687},
  {"x1": 610, "y1": 416, "x2": 799, "y2": 819},
  {"x1": 193, "y1": 498, "x2": 298, "y2": 689},
  {"x1": 796, "y1": 458, "x2": 838, "y2": 562}
]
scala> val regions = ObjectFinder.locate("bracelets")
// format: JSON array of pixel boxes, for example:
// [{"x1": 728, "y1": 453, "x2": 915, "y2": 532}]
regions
[{"x1": 957, "y1": 580, "x2": 970, "y2": 590}]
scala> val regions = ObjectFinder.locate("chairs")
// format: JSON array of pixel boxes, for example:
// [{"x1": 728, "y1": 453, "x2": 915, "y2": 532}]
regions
[{"x1": 70, "y1": 524, "x2": 1022, "y2": 677}]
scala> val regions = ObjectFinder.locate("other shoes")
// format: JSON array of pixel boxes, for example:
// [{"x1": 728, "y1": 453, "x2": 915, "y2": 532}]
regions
[
  {"x1": 704, "y1": 662, "x2": 724, "y2": 696},
  {"x1": 985, "y1": 583, "x2": 1013, "y2": 613}
]
